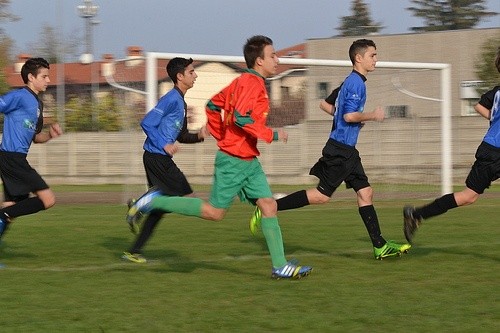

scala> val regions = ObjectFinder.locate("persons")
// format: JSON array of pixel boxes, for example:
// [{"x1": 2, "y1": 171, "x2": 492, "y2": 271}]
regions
[
  {"x1": 0, "y1": 57, "x2": 62, "y2": 239},
  {"x1": 121, "y1": 57, "x2": 211, "y2": 263},
  {"x1": 249, "y1": 39, "x2": 410, "y2": 260},
  {"x1": 405, "y1": 57, "x2": 500, "y2": 243},
  {"x1": 127, "y1": 35, "x2": 312, "y2": 280}
]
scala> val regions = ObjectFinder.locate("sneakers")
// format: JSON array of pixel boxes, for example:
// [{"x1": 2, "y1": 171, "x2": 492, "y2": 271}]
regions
[
  {"x1": 373, "y1": 240, "x2": 411, "y2": 260},
  {"x1": 249, "y1": 207, "x2": 262, "y2": 236},
  {"x1": 134, "y1": 186, "x2": 159, "y2": 219},
  {"x1": 271, "y1": 261, "x2": 312, "y2": 281}
]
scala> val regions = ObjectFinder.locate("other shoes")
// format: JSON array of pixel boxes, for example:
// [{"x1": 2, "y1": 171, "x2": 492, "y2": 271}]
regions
[
  {"x1": 120, "y1": 247, "x2": 146, "y2": 263},
  {"x1": 404, "y1": 207, "x2": 422, "y2": 244},
  {"x1": 128, "y1": 198, "x2": 143, "y2": 234}
]
